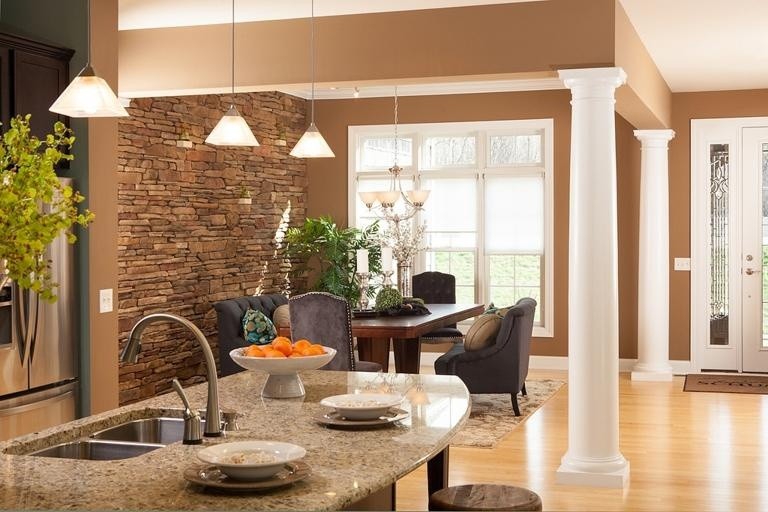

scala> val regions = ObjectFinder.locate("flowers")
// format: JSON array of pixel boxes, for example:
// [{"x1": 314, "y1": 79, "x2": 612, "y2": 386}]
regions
[{"x1": 367, "y1": 204, "x2": 436, "y2": 267}]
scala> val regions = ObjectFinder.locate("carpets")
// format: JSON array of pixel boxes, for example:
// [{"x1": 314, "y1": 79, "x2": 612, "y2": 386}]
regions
[
  {"x1": 682, "y1": 371, "x2": 768, "y2": 395},
  {"x1": 446, "y1": 375, "x2": 566, "y2": 447}
]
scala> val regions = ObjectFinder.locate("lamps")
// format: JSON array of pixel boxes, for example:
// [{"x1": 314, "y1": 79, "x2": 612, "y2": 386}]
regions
[
  {"x1": 45, "y1": 0, "x2": 130, "y2": 123},
  {"x1": 358, "y1": 81, "x2": 432, "y2": 212},
  {"x1": 203, "y1": 1, "x2": 262, "y2": 149},
  {"x1": 289, "y1": 2, "x2": 334, "y2": 160}
]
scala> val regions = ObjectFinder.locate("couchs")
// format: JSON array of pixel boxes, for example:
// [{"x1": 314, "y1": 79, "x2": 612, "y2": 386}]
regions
[
  {"x1": 214, "y1": 292, "x2": 290, "y2": 377},
  {"x1": 433, "y1": 294, "x2": 539, "y2": 417}
]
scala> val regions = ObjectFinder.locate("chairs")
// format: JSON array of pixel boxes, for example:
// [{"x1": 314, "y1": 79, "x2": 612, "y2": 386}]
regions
[
  {"x1": 408, "y1": 271, "x2": 465, "y2": 348},
  {"x1": 288, "y1": 288, "x2": 386, "y2": 375}
]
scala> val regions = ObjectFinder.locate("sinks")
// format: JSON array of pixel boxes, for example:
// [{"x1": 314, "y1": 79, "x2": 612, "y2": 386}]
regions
[
  {"x1": 90, "y1": 418, "x2": 224, "y2": 444},
  {"x1": 27, "y1": 441, "x2": 161, "y2": 460}
]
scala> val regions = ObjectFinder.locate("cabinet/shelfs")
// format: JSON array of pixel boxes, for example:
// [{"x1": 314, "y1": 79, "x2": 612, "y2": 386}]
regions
[{"x1": 0, "y1": 29, "x2": 80, "y2": 170}]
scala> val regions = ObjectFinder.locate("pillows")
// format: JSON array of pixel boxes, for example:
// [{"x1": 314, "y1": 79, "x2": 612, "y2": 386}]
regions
[
  {"x1": 240, "y1": 306, "x2": 278, "y2": 344},
  {"x1": 466, "y1": 309, "x2": 504, "y2": 348},
  {"x1": 272, "y1": 301, "x2": 292, "y2": 340}
]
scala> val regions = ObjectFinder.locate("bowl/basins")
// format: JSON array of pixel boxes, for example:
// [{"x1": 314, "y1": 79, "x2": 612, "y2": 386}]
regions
[
  {"x1": 193, "y1": 440, "x2": 306, "y2": 482},
  {"x1": 318, "y1": 390, "x2": 406, "y2": 420}
]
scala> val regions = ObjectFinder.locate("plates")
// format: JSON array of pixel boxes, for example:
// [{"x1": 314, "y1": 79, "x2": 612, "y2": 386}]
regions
[
  {"x1": 183, "y1": 461, "x2": 314, "y2": 495},
  {"x1": 317, "y1": 410, "x2": 410, "y2": 429}
]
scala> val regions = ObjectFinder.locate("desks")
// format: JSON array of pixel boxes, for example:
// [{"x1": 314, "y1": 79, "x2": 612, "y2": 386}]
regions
[{"x1": 274, "y1": 298, "x2": 487, "y2": 377}]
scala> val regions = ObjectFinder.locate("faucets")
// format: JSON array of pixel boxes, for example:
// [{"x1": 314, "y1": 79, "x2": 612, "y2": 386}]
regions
[{"x1": 121, "y1": 312, "x2": 225, "y2": 437}]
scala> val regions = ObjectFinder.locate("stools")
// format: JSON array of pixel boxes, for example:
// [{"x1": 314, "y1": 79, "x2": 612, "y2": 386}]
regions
[{"x1": 426, "y1": 478, "x2": 544, "y2": 510}]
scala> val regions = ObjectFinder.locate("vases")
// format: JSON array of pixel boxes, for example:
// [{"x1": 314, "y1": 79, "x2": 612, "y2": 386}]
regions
[{"x1": 398, "y1": 261, "x2": 412, "y2": 299}]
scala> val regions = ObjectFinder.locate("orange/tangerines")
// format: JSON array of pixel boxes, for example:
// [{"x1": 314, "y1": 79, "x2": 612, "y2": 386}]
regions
[{"x1": 241, "y1": 336, "x2": 324, "y2": 358}]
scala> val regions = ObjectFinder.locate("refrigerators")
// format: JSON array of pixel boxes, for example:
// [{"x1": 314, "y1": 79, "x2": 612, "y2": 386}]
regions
[{"x1": 1, "y1": 176, "x2": 80, "y2": 398}]
